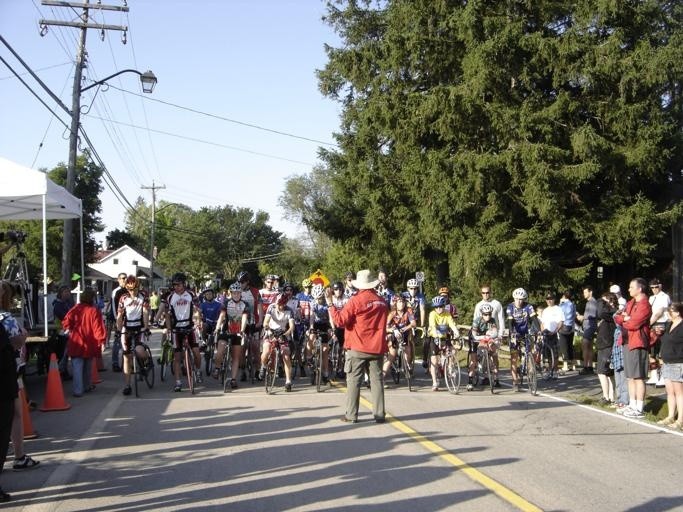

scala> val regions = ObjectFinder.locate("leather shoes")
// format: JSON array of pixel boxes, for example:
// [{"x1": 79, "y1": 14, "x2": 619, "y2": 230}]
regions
[
  {"x1": 340, "y1": 415, "x2": 357, "y2": 423},
  {"x1": 0, "y1": 491, "x2": 10, "y2": 502}
]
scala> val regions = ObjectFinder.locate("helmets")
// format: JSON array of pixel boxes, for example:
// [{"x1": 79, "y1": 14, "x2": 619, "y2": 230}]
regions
[
  {"x1": 125, "y1": 275, "x2": 140, "y2": 290},
  {"x1": 273, "y1": 274, "x2": 280, "y2": 280},
  {"x1": 202, "y1": 286, "x2": 214, "y2": 293},
  {"x1": 238, "y1": 270, "x2": 248, "y2": 282},
  {"x1": 479, "y1": 304, "x2": 493, "y2": 313},
  {"x1": 311, "y1": 283, "x2": 325, "y2": 299},
  {"x1": 392, "y1": 292, "x2": 405, "y2": 301},
  {"x1": 512, "y1": 287, "x2": 528, "y2": 300},
  {"x1": 439, "y1": 286, "x2": 449, "y2": 295},
  {"x1": 345, "y1": 271, "x2": 357, "y2": 280},
  {"x1": 302, "y1": 278, "x2": 313, "y2": 286},
  {"x1": 229, "y1": 283, "x2": 242, "y2": 292},
  {"x1": 276, "y1": 293, "x2": 289, "y2": 305},
  {"x1": 171, "y1": 273, "x2": 187, "y2": 282},
  {"x1": 333, "y1": 281, "x2": 344, "y2": 289},
  {"x1": 431, "y1": 295, "x2": 446, "y2": 307},
  {"x1": 406, "y1": 278, "x2": 420, "y2": 289},
  {"x1": 265, "y1": 274, "x2": 275, "y2": 280}
]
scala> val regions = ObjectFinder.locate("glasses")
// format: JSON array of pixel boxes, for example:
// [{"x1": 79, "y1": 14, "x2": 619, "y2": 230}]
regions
[
  {"x1": 267, "y1": 281, "x2": 274, "y2": 284},
  {"x1": 172, "y1": 283, "x2": 182, "y2": 286},
  {"x1": 335, "y1": 288, "x2": 342, "y2": 291},
  {"x1": 482, "y1": 291, "x2": 489, "y2": 294},
  {"x1": 546, "y1": 296, "x2": 554, "y2": 300},
  {"x1": 651, "y1": 286, "x2": 659, "y2": 289},
  {"x1": 484, "y1": 314, "x2": 491, "y2": 316},
  {"x1": 285, "y1": 288, "x2": 293, "y2": 292},
  {"x1": 305, "y1": 286, "x2": 311, "y2": 288}
]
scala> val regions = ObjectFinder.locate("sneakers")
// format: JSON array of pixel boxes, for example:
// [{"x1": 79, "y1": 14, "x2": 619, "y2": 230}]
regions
[
  {"x1": 259, "y1": 365, "x2": 266, "y2": 380},
  {"x1": 624, "y1": 409, "x2": 645, "y2": 418},
  {"x1": 212, "y1": 368, "x2": 220, "y2": 380},
  {"x1": 668, "y1": 420, "x2": 683, "y2": 428},
  {"x1": 123, "y1": 387, "x2": 132, "y2": 395},
  {"x1": 12, "y1": 454, "x2": 41, "y2": 472},
  {"x1": 174, "y1": 383, "x2": 182, "y2": 392},
  {"x1": 195, "y1": 370, "x2": 203, "y2": 383},
  {"x1": 466, "y1": 383, "x2": 473, "y2": 390},
  {"x1": 656, "y1": 417, "x2": 676, "y2": 425},
  {"x1": 579, "y1": 366, "x2": 594, "y2": 376},
  {"x1": 616, "y1": 405, "x2": 633, "y2": 415},
  {"x1": 230, "y1": 379, "x2": 238, "y2": 389},
  {"x1": 240, "y1": 371, "x2": 247, "y2": 382},
  {"x1": 284, "y1": 384, "x2": 291, "y2": 393},
  {"x1": 322, "y1": 376, "x2": 331, "y2": 386},
  {"x1": 382, "y1": 379, "x2": 389, "y2": 389},
  {"x1": 432, "y1": 383, "x2": 438, "y2": 391}
]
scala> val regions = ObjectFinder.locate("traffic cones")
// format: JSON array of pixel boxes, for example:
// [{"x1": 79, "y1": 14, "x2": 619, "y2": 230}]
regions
[
  {"x1": 96, "y1": 358, "x2": 106, "y2": 372},
  {"x1": 39, "y1": 354, "x2": 74, "y2": 415},
  {"x1": 89, "y1": 356, "x2": 104, "y2": 384},
  {"x1": 15, "y1": 372, "x2": 39, "y2": 445}
]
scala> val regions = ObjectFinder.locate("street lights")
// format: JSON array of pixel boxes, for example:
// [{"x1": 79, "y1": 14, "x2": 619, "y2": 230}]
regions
[{"x1": 58, "y1": 68, "x2": 158, "y2": 291}]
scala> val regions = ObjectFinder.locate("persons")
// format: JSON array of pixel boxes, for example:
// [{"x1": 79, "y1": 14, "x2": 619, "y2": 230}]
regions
[
  {"x1": 0, "y1": 281, "x2": 41, "y2": 473},
  {"x1": 1, "y1": 319, "x2": 20, "y2": 504},
  {"x1": 2, "y1": 269, "x2": 682, "y2": 432}
]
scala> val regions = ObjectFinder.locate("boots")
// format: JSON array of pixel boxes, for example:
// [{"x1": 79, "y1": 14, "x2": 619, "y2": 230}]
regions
[
  {"x1": 645, "y1": 369, "x2": 658, "y2": 384},
  {"x1": 656, "y1": 375, "x2": 666, "y2": 388}
]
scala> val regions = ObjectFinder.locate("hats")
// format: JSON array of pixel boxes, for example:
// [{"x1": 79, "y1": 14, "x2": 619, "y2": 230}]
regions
[
  {"x1": 351, "y1": 268, "x2": 381, "y2": 290},
  {"x1": 648, "y1": 277, "x2": 661, "y2": 285},
  {"x1": 609, "y1": 284, "x2": 621, "y2": 293},
  {"x1": 283, "y1": 282, "x2": 294, "y2": 291}
]
